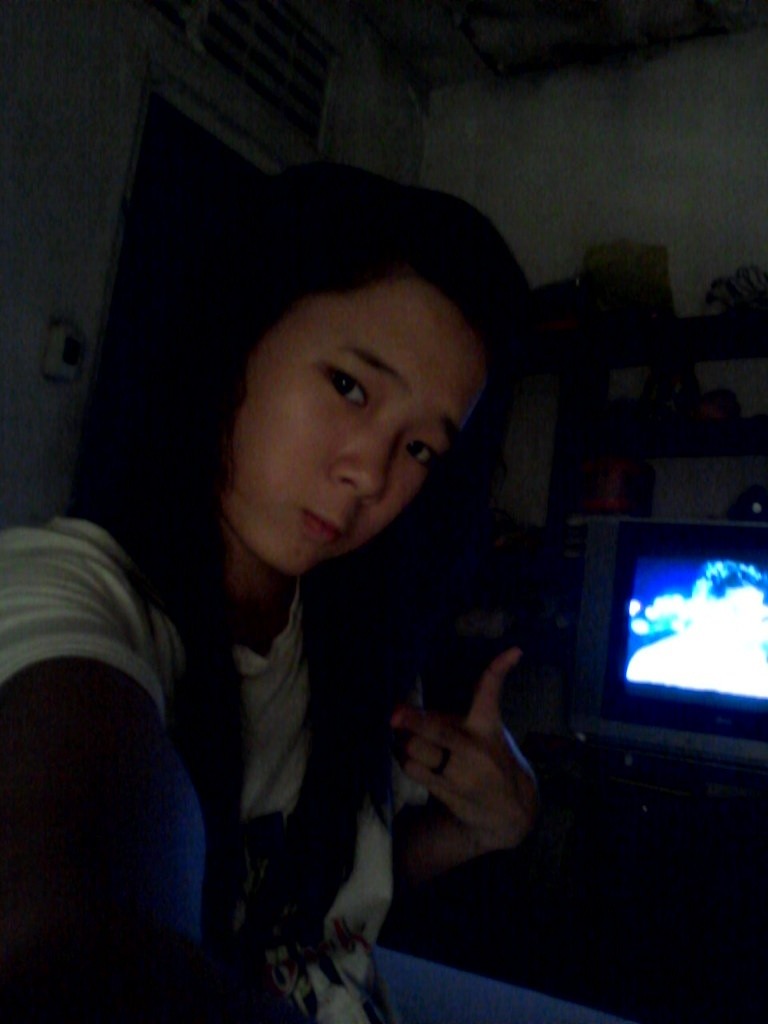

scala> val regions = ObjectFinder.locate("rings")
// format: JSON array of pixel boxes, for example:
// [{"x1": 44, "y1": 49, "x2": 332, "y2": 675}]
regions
[{"x1": 429, "y1": 747, "x2": 451, "y2": 774}]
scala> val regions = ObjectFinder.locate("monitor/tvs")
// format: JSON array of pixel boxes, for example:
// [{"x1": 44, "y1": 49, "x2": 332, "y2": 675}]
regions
[{"x1": 566, "y1": 517, "x2": 768, "y2": 770}]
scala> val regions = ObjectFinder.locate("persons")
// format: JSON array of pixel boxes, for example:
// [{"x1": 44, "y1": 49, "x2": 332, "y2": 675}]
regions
[{"x1": 0, "y1": 160, "x2": 642, "y2": 1024}]
